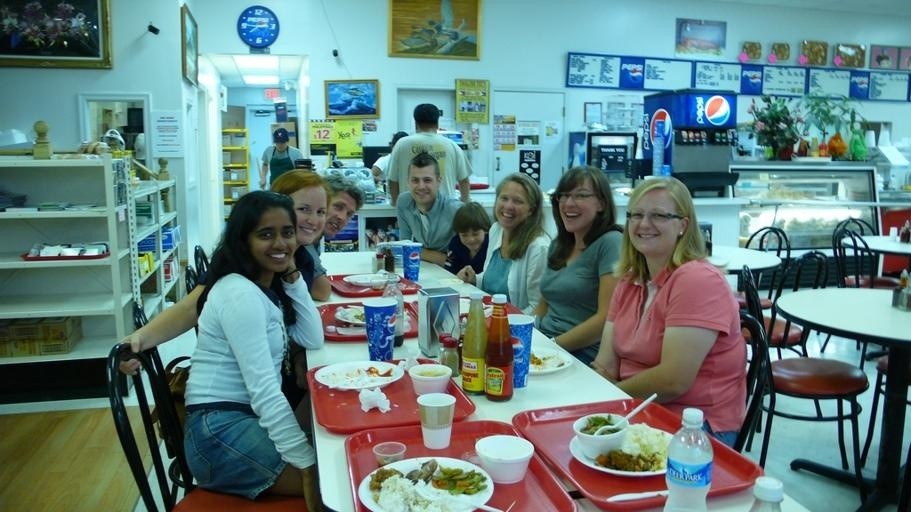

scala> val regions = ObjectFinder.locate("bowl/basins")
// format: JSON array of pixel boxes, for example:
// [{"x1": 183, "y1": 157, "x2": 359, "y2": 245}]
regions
[{"x1": 852, "y1": 191, "x2": 869, "y2": 202}]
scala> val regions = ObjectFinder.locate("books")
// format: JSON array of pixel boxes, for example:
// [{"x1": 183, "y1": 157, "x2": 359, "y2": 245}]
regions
[{"x1": 4, "y1": 200, "x2": 109, "y2": 213}]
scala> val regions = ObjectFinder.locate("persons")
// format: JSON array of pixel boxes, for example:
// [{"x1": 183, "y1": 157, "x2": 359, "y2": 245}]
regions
[
  {"x1": 589, "y1": 175, "x2": 747, "y2": 450},
  {"x1": 371, "y1": 130, "x2": 410, "y2": 183},
  {"x1": 258, "y1": 128, "x2": 305, "y2": 191},
  {"x1": 394, "y1": 151, "x2": 467, "y2": 267},
  {"x1": 180, "y1": 190, "x2": 317, "y2": 501},
  {"x1": 522, "y1": 165, "x2": 625, "y2": 368},
  {"x1": 456, "y1": 172, "x2": 554, "y2": 317},
  {"x1": 114, "y1": 168, "x2": 330, "y2": 388},
  {"x1": 443, "y1": 200, "x2": 493, "y2": 276},
  {"x1": 302, "y1": 169, "x2": 366, "y2": 302},
  {"x1": 387, "y1": 103, "x2": 473, "y2": 208}
]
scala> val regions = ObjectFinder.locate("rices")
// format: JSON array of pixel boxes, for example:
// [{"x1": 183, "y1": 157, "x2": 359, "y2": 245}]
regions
[
  {"x1": 378, "y1": 475, "x2": 460, "y2": 511},
  {"x1": 626, "y1": 422, "x2": 669, "y2": 455}
]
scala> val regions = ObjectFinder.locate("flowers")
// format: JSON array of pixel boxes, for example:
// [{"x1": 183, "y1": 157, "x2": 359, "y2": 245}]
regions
[{"x1": 747, "y1": 94, "x2": 806, "y2": 148}]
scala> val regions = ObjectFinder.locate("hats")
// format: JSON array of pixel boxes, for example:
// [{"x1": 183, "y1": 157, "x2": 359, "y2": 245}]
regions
[{"x1": 274, "y1": 128, "x2": 288, "y2": 143}]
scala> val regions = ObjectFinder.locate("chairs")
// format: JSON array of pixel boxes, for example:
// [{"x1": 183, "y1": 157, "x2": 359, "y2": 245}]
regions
[
  {"x1": 194, "y1": 246, "x2": 210, "y2": 277},
  {"x1": 105, "y1": 342, "x2": 306, "y2": 512},
  {"x1": 129, "y1": 303, "x2": 193, "y2": 496},
  {"x1": 185, "y1": 266, "x2": 198, "y2": 338},
  {"x1": 732, "y1": 219, "x2": 878, "y2": 512}
]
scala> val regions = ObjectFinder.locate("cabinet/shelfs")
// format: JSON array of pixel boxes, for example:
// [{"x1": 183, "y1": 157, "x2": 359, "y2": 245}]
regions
[
  {"x1": 728, "y1": 166, "x2": 883, "y2": 249},
  {"x1": 223, "y1": 127, "x2": 250, "y2": 221},
  {"x1": 0, "y1": 150, "x2": 182, "y2": 406}
]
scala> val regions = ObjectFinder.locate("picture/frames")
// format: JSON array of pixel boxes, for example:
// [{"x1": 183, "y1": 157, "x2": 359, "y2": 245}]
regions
[
  {"x1": 179, "y1": 2, "x2": 197, "y2": 88},
  {"x1": 388, "y1": 0, "x2": 480, "y2": 60},
  {"x1": 676, "y1": 18, "x2": 726, "y2": 58},
  {"x1": 0, "y1": 0, "x2": 112, "y2": 68}
]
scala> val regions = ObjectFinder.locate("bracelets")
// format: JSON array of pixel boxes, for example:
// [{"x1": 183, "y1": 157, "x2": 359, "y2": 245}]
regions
[{"x1": 549, "y1": 337, "x2": 558, "y2": 346}]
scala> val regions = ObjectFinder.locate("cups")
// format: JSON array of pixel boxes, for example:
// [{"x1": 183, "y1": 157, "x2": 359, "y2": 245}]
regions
[
  {"x1": 651, "y1": 119, "x2": 672, "y2": 178},
  {"x1": 416, "y1": 393, "x2": 456, "y2": 450},
  {"x1": 889, "y1": 227, "x2": 897, "y2": 241},
  {"x1": 475, "y1": 435, "x2": 535, "y2": 485},
  {"x1": 372, "y1": 441, "x2": 407, "y2": 468},
  {"x1": 402, "y1": 243, "x2": 422, "y2": 282},
  {"x1": 864, "y1": 130, "x2": 875, "y2": 147},
  {"x1": 573, "y1": 413, "x2": 629, "y2": 460},
  {"x1": 370, "y1": 279, "x2": 384, "y2": 291},
  {"x1": 408, "y1": 363, "x2": 452, "y2": 396},
  {"x1": 505, "y1": 312, "x2": 536, "y2": 389},
  {"x1": 362, "y1": 297, "x2": 398, "y2": 361}
]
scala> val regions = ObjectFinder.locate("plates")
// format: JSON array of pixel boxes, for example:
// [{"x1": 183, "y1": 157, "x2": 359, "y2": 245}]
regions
[
  {"x1": 342, "y1": 274, "x2": 401, "y2": 286},
  {"x1": 459, "y1": 298, "x2": 471, "y2": 317},
  {"x1": 334, "y1": 308, "x2": 365, "y2": 327},
  {"x1": 314, "y1": 360, "x2": 404, "y2": 390},
  {"x1": 569, "y1": 425, "x2": 674, "y2": 477},
  {"x1": 528, "y1": 347, "x2": 574, "y2": 375},
  {"x1": 358, "y1": 456, "x2": 495, "y2": 512}
]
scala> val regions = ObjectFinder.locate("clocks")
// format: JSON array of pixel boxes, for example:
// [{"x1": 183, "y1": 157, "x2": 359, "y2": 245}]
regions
[{"x1": 237, "y1": 5, "x2": 279, "y2": 49}]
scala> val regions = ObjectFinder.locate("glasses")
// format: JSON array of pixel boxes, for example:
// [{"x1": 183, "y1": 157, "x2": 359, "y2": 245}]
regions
[
  {"x1": 627, "y1": 208, "x2": 684, "y2": 223},
  {"x1": 555, "y1": 192, "x2": 597, "y2": 203}
]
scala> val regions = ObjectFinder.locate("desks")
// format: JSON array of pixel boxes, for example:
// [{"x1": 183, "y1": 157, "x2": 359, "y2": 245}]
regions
[
  {"x1": 777, "y1": 288, "x2": 910, "y2": 512},
  {"x1": 841, "y1": 235, "x2": 910, "y2": 286}
]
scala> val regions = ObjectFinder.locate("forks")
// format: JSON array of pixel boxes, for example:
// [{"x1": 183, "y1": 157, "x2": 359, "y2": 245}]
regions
[
  {"x1": 591, "y1": 393, "x2": 659, "y2": 435},
  {"x1": 414, "y1": 481, "x2": 505, "y2": 512}
]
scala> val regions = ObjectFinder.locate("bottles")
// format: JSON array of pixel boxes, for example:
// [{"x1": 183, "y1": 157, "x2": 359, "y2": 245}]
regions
[
  {"x1": 383, "y1": 275, "x2": 405, "y2": 348},
  {"x1": 891, "y1": 268, "x2": 911, "y2": 311},
  {"x1": 437, "y1": 333, "x2": 459, "y2": 377},
  {"x1": 461, "y1": 297, "x2": 486, "y2": 395},
  {"x1": 376, "y1": 253, "x2": 384, "y2": 274},
  {"x1": 899, "y1": 221, "x2": 911, "y2": 243},
  {"x1": 747, "y1": 477, "x2": 785, "y2": 512},
  {"x1": 384, "y1": 249, "x2": 395, "y2": 272},
  {"x1": 704, "y1": 230, "x2": 712, "y2": 255},
  {"x1": 797, "y1": 136, "x2": 829, "y2": 157},
  {"x1": 484, "y1": 293, "x2": 514, "y2": 403},
  {"x1": 371, "y1": 255, "x2": 377, "y2": 274},
  {"x1": 660, "y1": 408, "x2": 714, "y2": 512}
]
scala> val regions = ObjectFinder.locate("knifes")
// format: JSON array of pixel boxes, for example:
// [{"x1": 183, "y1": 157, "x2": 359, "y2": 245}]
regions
[{"x1": 607, "y1": 489, "x2": 669, "y2": 502}]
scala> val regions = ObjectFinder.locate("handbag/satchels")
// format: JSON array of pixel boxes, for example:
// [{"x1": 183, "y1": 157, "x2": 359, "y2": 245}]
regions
[{"x1": 151, "y1": 356, "x2": 191, "y2": 438}]
